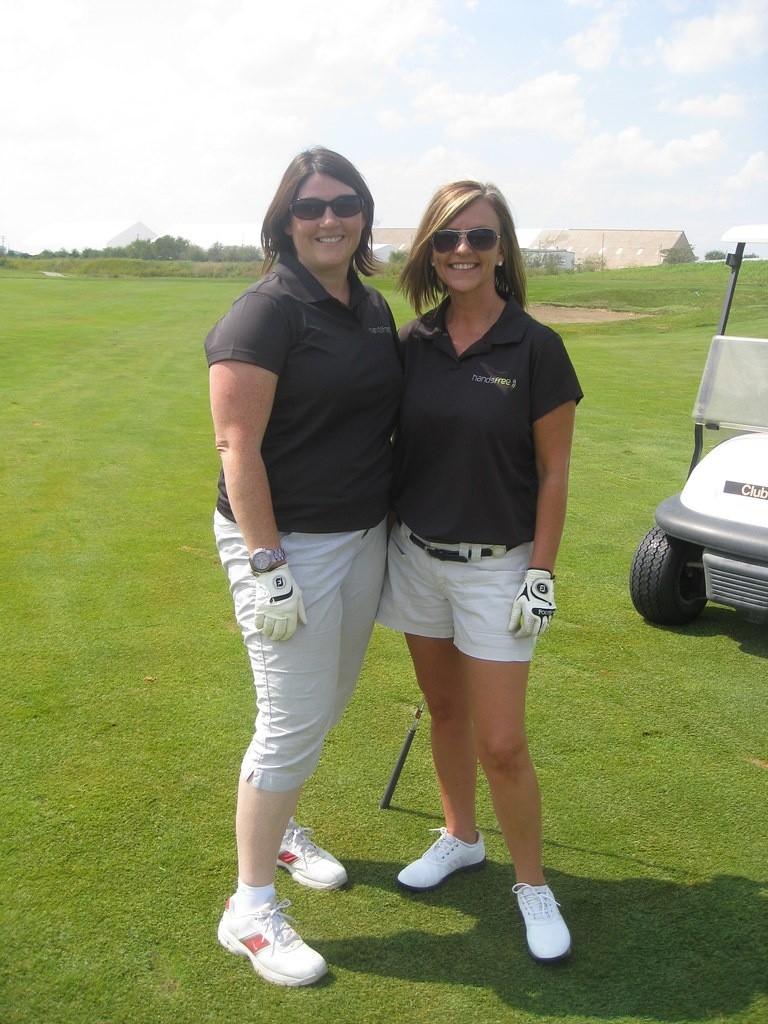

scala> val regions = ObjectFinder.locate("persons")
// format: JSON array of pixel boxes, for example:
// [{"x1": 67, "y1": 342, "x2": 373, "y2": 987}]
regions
[
  {"x1": 375, "y1": 181, "x2": 584, "y2": 964},
  {"x1": 206, "y1": 146, "x2": 401, "y2": 986}
]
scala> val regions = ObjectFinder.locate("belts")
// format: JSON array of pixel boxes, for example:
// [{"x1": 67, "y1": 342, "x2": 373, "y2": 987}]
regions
[{"x1": 394, "y1": 518, "x2": 492, "y2": 563}]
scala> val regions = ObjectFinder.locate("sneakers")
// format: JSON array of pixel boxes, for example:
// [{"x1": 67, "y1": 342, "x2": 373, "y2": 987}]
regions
[
  {"x1": 396, "y1": 827, "x2": 487, "y2": 891},
  {"x1": 276, "y1": 815, "x2": 348, "y2": 890},
  {"x1": 217, "y1": 893, "x2": 328, "y2": 987},
  {"x1": 511, "y1": 882, "x2": 572, "y2": 962}
]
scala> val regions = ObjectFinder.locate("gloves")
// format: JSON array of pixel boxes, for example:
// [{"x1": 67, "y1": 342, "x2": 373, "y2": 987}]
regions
[
  {"x1": 251, "y1": 562, "x2": 307, "y2": 642},
  {"x1": 508, "y1": 568, "x2": 557, "y2": 639}
]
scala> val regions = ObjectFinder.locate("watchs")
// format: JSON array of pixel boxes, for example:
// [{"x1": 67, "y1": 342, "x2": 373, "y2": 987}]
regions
[{"x1": 249, "y1": 547, "x2": 285, "y2": 573}]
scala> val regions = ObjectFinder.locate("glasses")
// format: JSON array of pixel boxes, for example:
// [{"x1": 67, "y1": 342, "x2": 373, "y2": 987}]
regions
[
  {"x1": 429, "y1": 227, "x2": 501, "y2": 253},
  {"x1": 289, "y1": 194, "x2": 364, "y2": 220}
]
